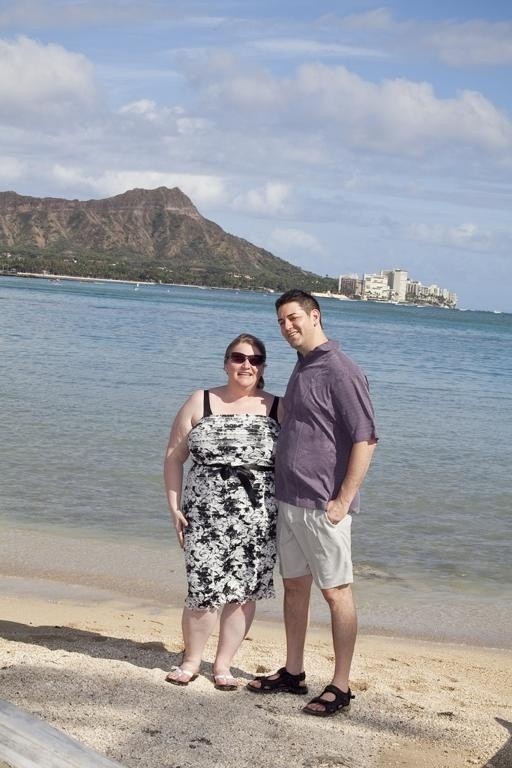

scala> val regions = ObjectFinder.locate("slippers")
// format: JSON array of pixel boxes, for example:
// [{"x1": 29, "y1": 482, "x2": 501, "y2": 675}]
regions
[
  {"x1": 214, "y1": 674, "x2": 240, "y2": 691},
  {"x1": 167, "y1": 667, "x2": 197, "y2": 685}
]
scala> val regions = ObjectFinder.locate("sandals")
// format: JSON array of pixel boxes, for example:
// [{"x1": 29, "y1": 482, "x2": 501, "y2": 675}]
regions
[
  {"x1": 247, "y1": 667, "x2": 307, "y2": 694},
  {"x1": 302, "y1": 686, "x2": 355, "y2": 716}
]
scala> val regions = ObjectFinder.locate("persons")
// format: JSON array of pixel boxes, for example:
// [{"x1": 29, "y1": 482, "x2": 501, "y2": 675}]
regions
[
  {"x1": 244, "y1": 287, "x2": 379, "y2": 715},
  {"x1": 164, "y1": 330, "x2": 288, "y2": 690}
]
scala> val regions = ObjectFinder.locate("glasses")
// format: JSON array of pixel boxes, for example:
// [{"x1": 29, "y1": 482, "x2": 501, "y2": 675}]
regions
[{"x1": 228, "y1": 351, "x2": 266, "y2": 366}]
